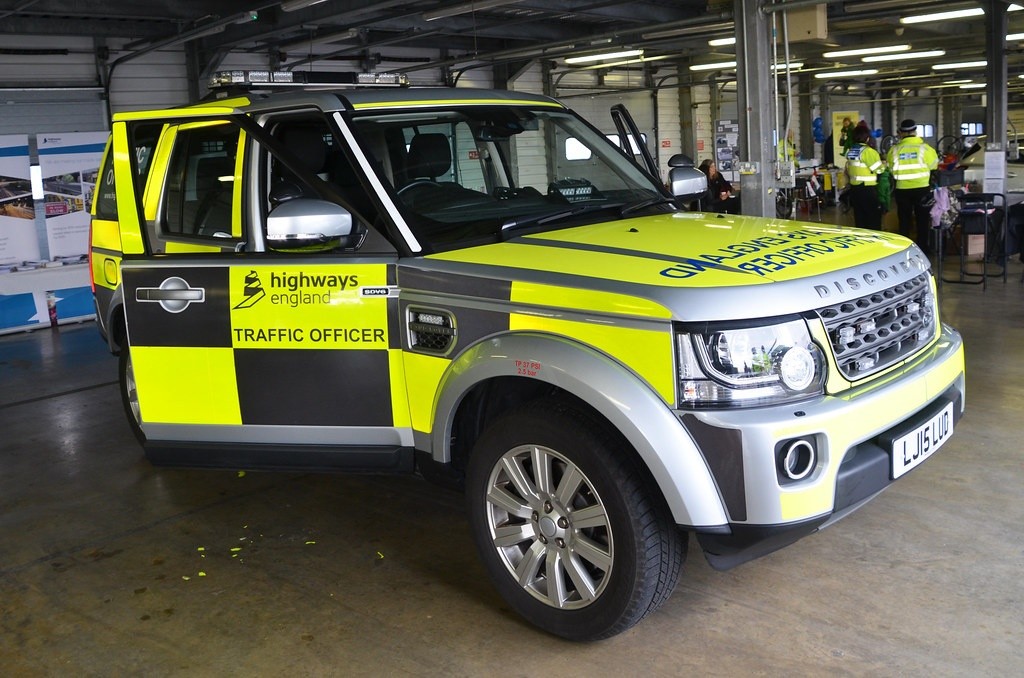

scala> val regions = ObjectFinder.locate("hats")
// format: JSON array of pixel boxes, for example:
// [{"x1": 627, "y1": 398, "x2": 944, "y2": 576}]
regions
[{"x1": 898, "y1": 119, "x2": 917, "y2": 132}]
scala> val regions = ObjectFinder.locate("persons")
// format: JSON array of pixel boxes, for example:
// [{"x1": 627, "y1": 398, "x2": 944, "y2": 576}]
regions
[
  {"x1": 840, "y1": 118, "x2": 854, "y2": 157},
  {"x1": 885, "y1": 119, "x2": 939, "y2": 254},
  {"x1": 699, "y1": 159, "x2": 733, "y2": 212},
  {"x1": 824, "y1": 130, "x2": 834, "y2": 168},
  {"x1": 846, "y1": 120, "x2": 886, "y2": 229},
  {"x1": 779, "y1": 129, "x2": 800, "y2": 168}
]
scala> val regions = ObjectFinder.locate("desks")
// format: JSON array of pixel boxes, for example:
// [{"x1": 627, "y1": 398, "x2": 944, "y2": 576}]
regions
[{"x1": 920, "y1": 193, "x2": 1024, "y2": 262}]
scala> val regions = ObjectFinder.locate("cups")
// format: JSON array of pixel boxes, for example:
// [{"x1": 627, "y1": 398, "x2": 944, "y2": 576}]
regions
[{"x1": 720, "y1": 192, "x2": 726, "y2": 200}]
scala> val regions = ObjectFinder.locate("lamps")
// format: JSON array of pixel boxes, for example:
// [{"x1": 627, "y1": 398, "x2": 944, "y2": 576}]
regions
[{"x1": 565, "y1": 1, "x2": 1024, "y2": 88}]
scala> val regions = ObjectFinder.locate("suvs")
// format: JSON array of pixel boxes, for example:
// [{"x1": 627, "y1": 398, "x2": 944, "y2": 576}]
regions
[{"x1": 88, "y1": 71, "x2": 966, "y2": 643}]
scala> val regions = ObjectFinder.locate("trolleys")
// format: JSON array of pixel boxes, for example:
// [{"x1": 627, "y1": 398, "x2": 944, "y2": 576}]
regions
[{"x1": 935, "y1": 193, "x2": 1008, "y2": 293}]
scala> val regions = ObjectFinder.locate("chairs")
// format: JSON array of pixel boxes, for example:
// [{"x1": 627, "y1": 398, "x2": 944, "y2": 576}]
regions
[
  {"x1": 190, "y1": 156, "x2": 233, "y2": 234},
  {"x1": 794, "y1": 178, "x2": 821, "y2": 222},
  {"x1": 403, "y1": 133, "x2": 464, "y2": 190},
  {"x1": 278, "y1": 132, "x2": 337, "y2": 179}
]
